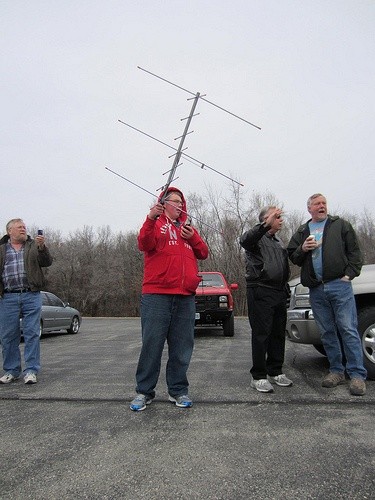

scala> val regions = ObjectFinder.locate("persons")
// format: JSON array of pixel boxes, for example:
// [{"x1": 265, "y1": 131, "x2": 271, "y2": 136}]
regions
[
  {"x1": 287, "y1": 193, "x2": 368, "y2": 396},
  {"x1": 239, "y1": 206, "x2": 293, "y2": 393},
  {"x1": 128, "y1": 187, "x2": 208, "y2": 412},
  {"x1": 0, "y1": 218, "x2": 53, "y2": 384}
]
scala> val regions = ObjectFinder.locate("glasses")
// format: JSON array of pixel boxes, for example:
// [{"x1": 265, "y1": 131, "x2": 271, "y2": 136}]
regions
[{"x1": 166, "y1": 199, "x2": 182, "y2": 204}]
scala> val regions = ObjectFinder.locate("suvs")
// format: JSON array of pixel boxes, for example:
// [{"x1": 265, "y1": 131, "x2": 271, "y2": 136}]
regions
[
  {"x1": 285, "y1": 263, "x2": 375, "y2": 378},
  {"x1": 194, "y1": 271, "x2": 239, "y2": 337}
]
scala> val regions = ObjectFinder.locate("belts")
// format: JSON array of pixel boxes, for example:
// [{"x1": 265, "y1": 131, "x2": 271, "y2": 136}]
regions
[{"x1": 3, "y1": 288, "x2": 31, "y2": 293}]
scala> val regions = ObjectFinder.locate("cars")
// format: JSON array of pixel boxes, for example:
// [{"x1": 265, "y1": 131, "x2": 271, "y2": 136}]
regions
[{"x1": 19, "y1": 290, "x2": 82, "y2": 338}]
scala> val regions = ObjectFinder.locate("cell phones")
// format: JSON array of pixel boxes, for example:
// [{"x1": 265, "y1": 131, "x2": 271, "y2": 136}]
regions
[{"x1": 38, "y1": 230, "x2": 43, "y2": 235}]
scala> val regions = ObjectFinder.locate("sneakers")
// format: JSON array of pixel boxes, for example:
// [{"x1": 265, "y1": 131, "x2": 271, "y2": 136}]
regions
[
  {"x1": 168, "y1": 393, "x2": 193, "y2": 408},
  {"x1": 348, "y1": 377, "x2": 366, "y2": 396},
  {"x1": 0, "y1": 372, "x2": 21, "y2": 384},
  {"x1": 250, "y1": 377, "x2": 274, "y2": 393},
  {"x1": 23, "y1": 372, "x2": 38, "y2": 384},
  {"x1": 322, "y1": 371, "x2": 345, "y2": 388},
  {"x1": 130, "y1": 393, "x2": 155, "y2": 411},
  {"x1": 270, "y1": 374, "x2": 293, "y2": 387}
]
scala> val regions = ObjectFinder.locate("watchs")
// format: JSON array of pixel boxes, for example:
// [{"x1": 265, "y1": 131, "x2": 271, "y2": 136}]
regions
[{"x1": 344, "y1": 274, "x2": 352, "y2": 281}]
